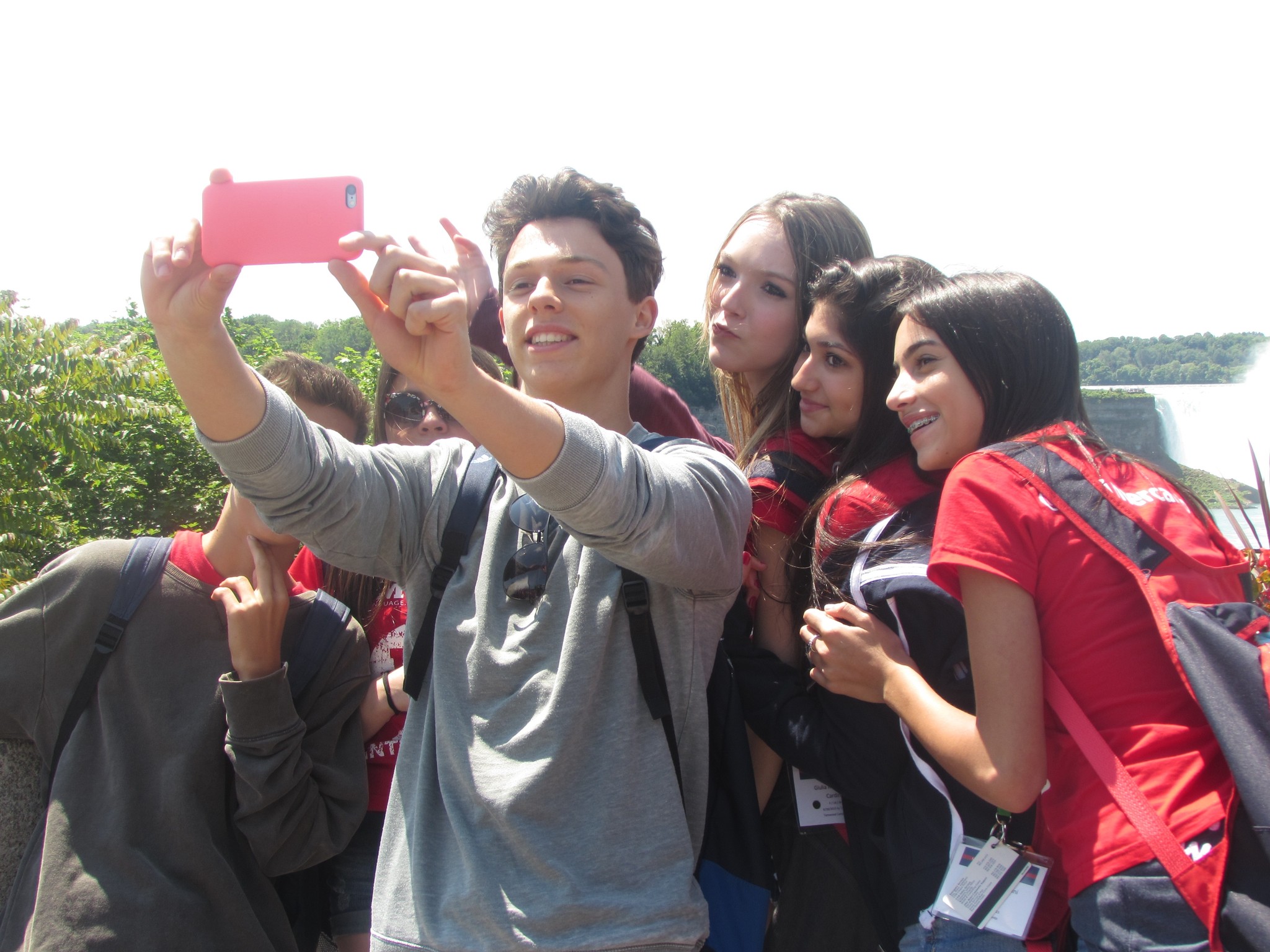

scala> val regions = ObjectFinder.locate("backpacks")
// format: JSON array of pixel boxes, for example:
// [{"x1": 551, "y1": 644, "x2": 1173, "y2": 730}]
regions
[{"x1": 953, "y1": 437, "x2": 1269, "y2": 952}]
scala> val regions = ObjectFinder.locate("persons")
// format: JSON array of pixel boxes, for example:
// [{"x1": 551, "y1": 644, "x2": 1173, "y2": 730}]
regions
[{"x1": 0, "y1": 168, "x2": 1270, "y2": 952}]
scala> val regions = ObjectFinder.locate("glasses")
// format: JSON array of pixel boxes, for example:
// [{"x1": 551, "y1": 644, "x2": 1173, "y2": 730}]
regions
[
  {"x1": 502, "y1": 493, "x2": 551, "y2": 605},
  {"x1": 384, "y1": 392, "x2": 464, "y2": 432}
]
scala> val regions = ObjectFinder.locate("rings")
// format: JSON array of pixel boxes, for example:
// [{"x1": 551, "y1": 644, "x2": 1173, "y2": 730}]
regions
[{"x1": 808, "y1": 633, "x2": 821, "y2": 653}]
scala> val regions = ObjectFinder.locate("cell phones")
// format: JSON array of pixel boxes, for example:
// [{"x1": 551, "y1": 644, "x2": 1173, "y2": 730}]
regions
[{"x1": 201, "y1": 176, "x2": 365, "y2": 265}]
